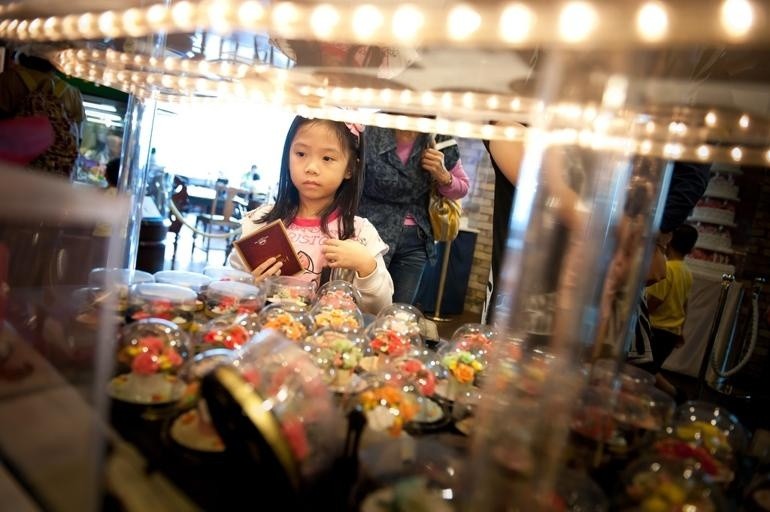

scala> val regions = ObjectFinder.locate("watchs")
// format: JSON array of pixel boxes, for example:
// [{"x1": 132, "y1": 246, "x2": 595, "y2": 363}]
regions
[{"x1": 437, "y1": 172, "x2": 454, "y2": 190}]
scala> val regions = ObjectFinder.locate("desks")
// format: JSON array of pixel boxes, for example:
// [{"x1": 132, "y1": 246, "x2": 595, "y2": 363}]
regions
[{"x1": 167, "y1": 183, "x2": 244, "y2": 240}]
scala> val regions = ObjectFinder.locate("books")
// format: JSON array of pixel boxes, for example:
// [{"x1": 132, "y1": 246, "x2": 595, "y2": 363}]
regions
[{"x1": 233, "y1": 218, "x2": 303, "y2": 277}]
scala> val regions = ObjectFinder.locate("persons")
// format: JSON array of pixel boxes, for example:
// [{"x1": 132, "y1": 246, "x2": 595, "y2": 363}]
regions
[
  {"x1": 241, "y1": 166, "x2": 257, "y2": 188},
  {"x1": 0, "y1": 47, "x2": 89, "y2": 178},
  {"x1": 250, "y1": 173, "x2": 267, "y2": 202},
  {"x1": 351, "y1": 125, "x2": 469, "y2": 308},
  {"x1": 227, "y1": 113, "x2": 394, "y2": 313},
  {"x1": 92, "y1": 159, "x2": 131, "y2": 254},
  {"x1": 647, "y1": 225, "x2": 700, "y2": 366},
  {"x1": 484, "y1": 118, "x2": 591, "y2": 350},
  {"x1": 149, "y1": 147, "x2": 156, "y2": 165}
]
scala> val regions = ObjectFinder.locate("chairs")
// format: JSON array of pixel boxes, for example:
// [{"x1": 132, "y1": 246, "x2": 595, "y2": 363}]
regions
[
  {"x1": 249, "y1": 193, "x2": 276, "y2": 214},
  {"x1": 148, "y1": 174, "x2": 187, "y2": 268},
  {"x1": 192, "y1": 186, "x2": 248, "y2": 266}
]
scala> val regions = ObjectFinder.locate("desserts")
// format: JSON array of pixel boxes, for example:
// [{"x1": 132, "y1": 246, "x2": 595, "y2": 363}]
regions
[{"x1": 65, "y1": 267, "x2": 770, "y2": 512}]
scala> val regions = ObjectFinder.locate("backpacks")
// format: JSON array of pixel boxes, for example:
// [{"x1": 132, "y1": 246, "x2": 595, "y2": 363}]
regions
[
  {"x1": 661, "y1": 165, "x2": 709, "y2": 234},
  {"x1": 14, "y1": 71, "x2": 82, "y2": 177}
]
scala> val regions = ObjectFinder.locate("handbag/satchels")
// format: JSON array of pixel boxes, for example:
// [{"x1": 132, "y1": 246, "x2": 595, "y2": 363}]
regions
[{"x1": 427, "y1": 141, "x2": 464, "y2": 243}]
